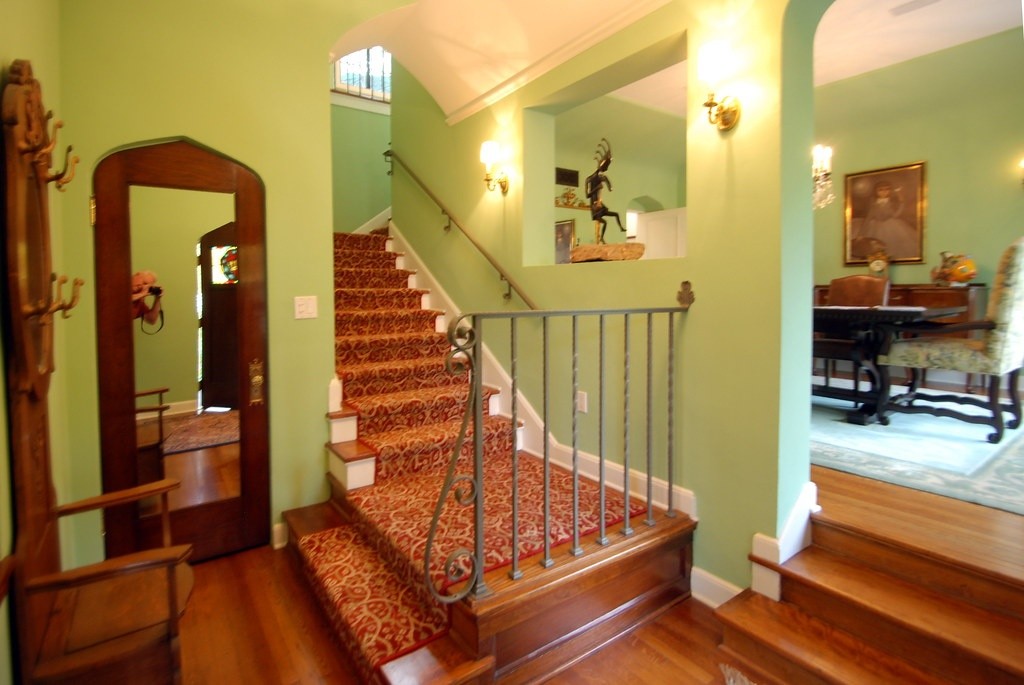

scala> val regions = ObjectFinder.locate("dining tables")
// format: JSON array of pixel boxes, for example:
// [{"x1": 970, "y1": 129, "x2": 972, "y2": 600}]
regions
[{"x1": 812, "y1": 304, "x2": 928, "y2": 428}]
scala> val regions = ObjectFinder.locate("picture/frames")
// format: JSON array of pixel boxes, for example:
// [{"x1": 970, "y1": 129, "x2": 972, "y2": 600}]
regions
[{"x1": 842, "y1": 159, "x2": 930, "y2": 266}]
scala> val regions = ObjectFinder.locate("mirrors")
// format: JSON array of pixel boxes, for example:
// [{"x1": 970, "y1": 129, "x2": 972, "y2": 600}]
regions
[{"x1": 125, "y1": 185, "x2": 245, "y2": 522}]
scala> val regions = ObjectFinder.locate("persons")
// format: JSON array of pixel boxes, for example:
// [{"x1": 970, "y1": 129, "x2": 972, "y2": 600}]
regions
[
  {"x1": 132, "y1": 269, "x2": 161, "y2": 326},
  {"x1": 584, "y1": 137, "x2": 626, "y2": 244}
]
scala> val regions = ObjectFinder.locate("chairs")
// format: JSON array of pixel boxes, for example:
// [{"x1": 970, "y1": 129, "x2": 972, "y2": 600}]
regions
[
  {"x1": 821, "y1": 274, "x2": 891, "y2": 407},
  {"x1": 0, "y1": 477, "x2": 201, "y2": 685},
  {"x1": 877, "y1": 234, "x2": 1024, "y2": 443},
  {"x1": 134, "y1": 386, "x2": 171, "y2": 516}
]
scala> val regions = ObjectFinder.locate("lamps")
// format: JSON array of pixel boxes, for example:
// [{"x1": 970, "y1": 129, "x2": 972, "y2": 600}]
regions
[
  {"x1": 697, "y1": 40, "x2": 740, "y2": 132},
  {"x1": 479, "y1": 140, "x2": 510, "y2": 196},
  {"x1": 811, "y1": 144, "x2": 836, "y2": 214}
]
